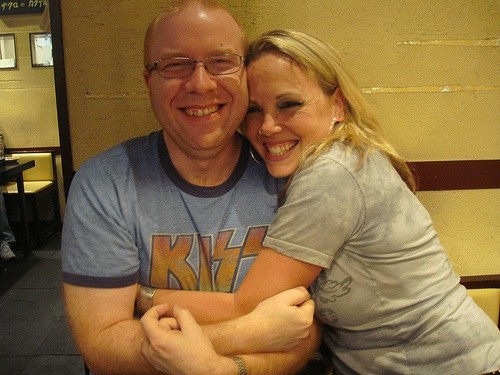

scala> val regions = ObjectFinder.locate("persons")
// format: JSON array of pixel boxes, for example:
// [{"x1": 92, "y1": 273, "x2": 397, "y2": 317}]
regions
[
  {"x1": 61, "y1": 0, "x2": 322, "y2": 375},
  {"x1": 0, "y1": 133, "x2": 16, "y2": 260},
  {"x1": 134, "y1": 28, "x2": 500, "y2": 375}
]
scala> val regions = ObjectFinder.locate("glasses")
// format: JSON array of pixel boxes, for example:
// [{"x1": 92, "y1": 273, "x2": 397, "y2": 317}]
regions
[{"x1": 148, "y1": 54, "x2": 247, "y2": 78}]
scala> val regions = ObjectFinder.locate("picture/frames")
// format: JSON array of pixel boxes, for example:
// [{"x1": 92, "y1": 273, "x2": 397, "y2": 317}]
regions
[
  {"x1": 0, "y1": 33, "x2": 17, "y2": 69},
  {"x1": 29, "y1": 32, "x2": 54, "y2": 67}
]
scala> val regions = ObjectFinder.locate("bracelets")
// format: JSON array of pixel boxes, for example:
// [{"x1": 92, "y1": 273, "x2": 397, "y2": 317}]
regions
[
  {"x1": 228, "y1": 354, "x2": 249, "y2": 375},
  {"x1": 138, "y1": 284, "x2": 158, "y2": 317}
]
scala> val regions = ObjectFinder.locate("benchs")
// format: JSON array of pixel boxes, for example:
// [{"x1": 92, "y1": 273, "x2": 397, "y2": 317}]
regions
[{"x1": 0, "y1": 147, "x2": 61, "y2": 248}]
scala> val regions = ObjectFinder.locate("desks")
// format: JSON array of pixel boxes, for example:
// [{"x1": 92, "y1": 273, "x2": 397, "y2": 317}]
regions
[{"x1": 0, "y1": 160, "x2": 35, "y2": 248}]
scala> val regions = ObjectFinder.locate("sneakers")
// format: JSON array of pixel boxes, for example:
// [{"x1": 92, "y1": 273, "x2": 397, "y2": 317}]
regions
[{"x1": 0, "y1": 244, "x2": 16, "y2": 261}]
